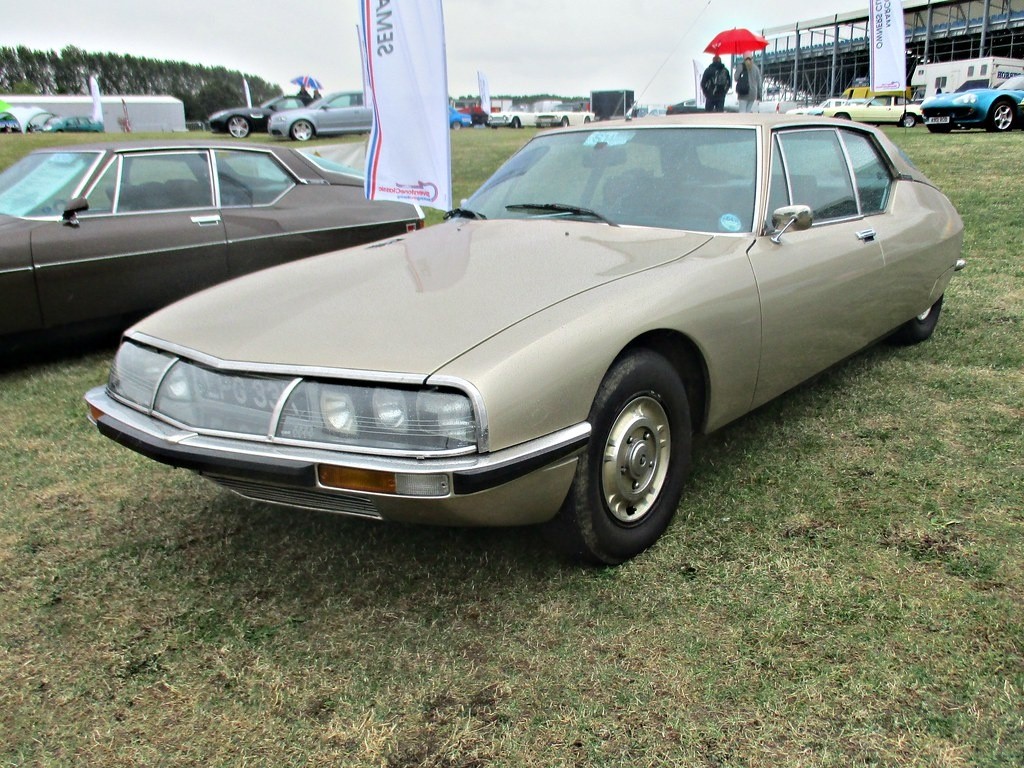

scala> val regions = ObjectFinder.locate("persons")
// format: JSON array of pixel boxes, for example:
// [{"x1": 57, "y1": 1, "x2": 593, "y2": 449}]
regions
[
  {"x1": 776, "y1": 103, "x2": 780, "y2": 114},
  {"x1": 733, "y1": 50, "x2": 761, "y2": 113},
  {"x1": 313, "y1": 90, "x2": 321, "y2": 100},
  {"x1": 700, "y1": 55, "x2": 731, "y2": 113},
  {"x1": 297, "y1": 85, "x2": 312, "y2": 103}
]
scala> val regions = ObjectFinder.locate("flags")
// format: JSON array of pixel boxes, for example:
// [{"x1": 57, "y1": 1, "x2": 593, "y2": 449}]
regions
[
  {"x1": 89, "y1": 77, "x2": 103, "y2": 121},
  {"x1": 357, "y1": 0, "x2": 452, "y2": 211},
  {"x1": 869, "y1": 0, "x2": 906, "y2": 92},
  {"x1": 478, "y1": 73, "x2": 490, "y2": 115}
]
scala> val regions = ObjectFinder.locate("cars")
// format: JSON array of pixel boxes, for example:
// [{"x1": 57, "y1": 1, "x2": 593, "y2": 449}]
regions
[
  {"x1": 208, "y1": 93, "x2": 329, "y2": 139},
  {"x1": 0, "y1": 110, "x2": 104, "y2": 133},
  {"x1": 0, "y1": 137, "x2": 426, "y2": 359},
  {"x1": 785, "y1": 56, "x2": 1024, "y2": 134},
  {"x1": 664, "y1": 99, "x2": 739, "y2": 115},
  {"x1": 486, "y1": 103, "x2": 541, "y2": 130},
  {"x1": 536, "y1": 102, "x2": 595, "y2": 130},
  {"x1": 265, "y1": 90, "x2": 374, "y2": 143},
  {"x1": 82, "y1": 111, "x2": 967, "y2": 569},
  {"x1": 448, "y1": 104, "x2": 472, "y2": 131}
]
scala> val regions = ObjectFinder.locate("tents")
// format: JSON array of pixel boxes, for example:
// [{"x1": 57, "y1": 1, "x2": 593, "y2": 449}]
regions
[{"x1": 0, "y1": 106, "x2": 55, "y2": 135}]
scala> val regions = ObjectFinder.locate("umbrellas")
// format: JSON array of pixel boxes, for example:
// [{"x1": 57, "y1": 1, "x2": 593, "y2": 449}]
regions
[
  {"x1": 702, "y1": 26, "x2": 770, "y2": 82},
  {"x1": 291, "y1": 74, "x2": 325, "y2": 91}
]
